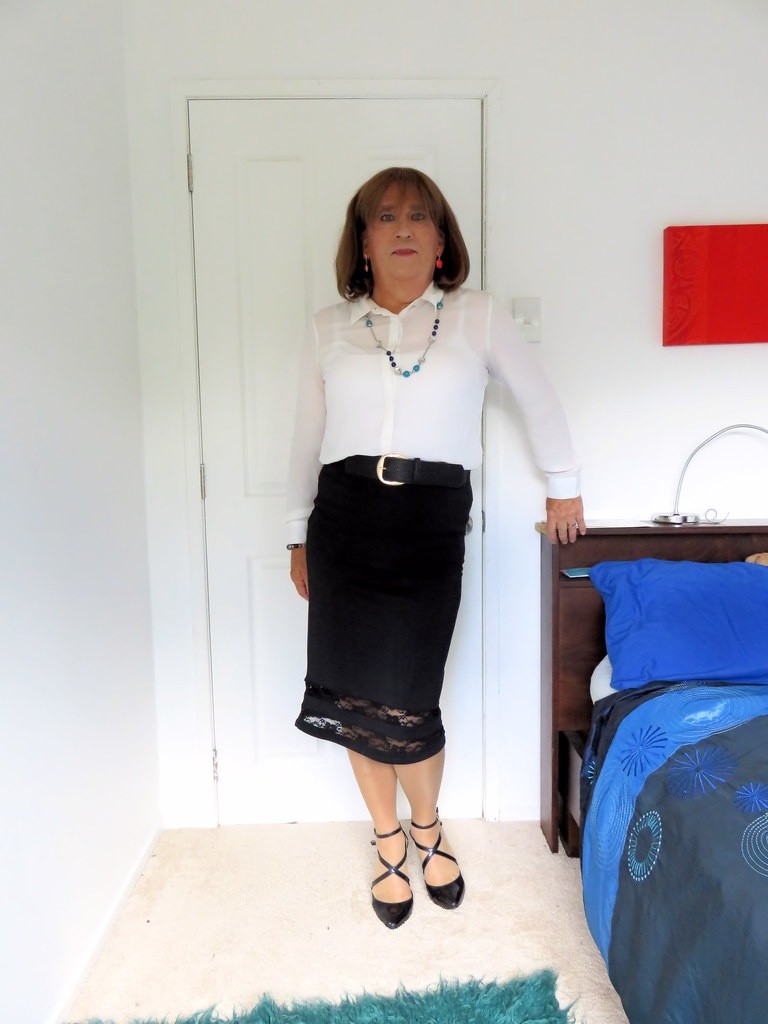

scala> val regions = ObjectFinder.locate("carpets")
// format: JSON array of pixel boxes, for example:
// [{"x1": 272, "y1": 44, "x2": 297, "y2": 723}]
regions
[{"x1": 61, "y1": 968, "x2": 586, "y2": 1024}]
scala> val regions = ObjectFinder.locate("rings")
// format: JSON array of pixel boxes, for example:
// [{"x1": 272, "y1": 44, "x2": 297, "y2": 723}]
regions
[{"x1": 567, "y1": 520, "x2": 578, "y2": 528}]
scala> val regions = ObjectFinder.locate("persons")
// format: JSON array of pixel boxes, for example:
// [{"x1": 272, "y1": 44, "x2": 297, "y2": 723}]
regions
[{"x1": 284, "y1": 168, "x2": 587, "y2": 930}]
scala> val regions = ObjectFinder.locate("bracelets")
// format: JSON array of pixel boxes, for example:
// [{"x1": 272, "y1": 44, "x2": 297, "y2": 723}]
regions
[{"x1": 286, "y1": 543, "x2": 306, "y2": 550}]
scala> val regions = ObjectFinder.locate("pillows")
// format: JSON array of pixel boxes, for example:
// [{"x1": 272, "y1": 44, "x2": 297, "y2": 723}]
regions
[{"x1": 590, "y1": 558, "x2": 768, "y2": 692}]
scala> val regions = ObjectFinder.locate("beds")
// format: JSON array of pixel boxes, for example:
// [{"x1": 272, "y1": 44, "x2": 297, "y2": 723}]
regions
[{"x1": 561, "y1": 655, "x2": 768, "y2": 1024}]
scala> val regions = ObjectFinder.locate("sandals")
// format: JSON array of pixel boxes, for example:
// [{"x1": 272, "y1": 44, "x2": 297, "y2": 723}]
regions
[
  {"x1": 371, "y1": 822, "x2": 413, "y2": 929},
  {"x1": 409, "y1": 807, "x2": 465, "y2": 909}
]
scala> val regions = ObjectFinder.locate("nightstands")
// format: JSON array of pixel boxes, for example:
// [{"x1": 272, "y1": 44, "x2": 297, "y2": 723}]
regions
[{"x1": 534, "y1": 517, "x2": 768, "y2": 859}]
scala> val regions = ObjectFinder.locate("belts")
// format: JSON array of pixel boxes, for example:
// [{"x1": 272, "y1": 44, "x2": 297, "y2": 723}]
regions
[{"x1": 322, "y1": 455, "x2": 470, "y2": 489}]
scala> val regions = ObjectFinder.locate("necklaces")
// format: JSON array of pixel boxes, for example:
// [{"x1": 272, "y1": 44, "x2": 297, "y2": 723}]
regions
[{"x1": 365, "y1": 299, "x2": 443, "y2": 377}]
